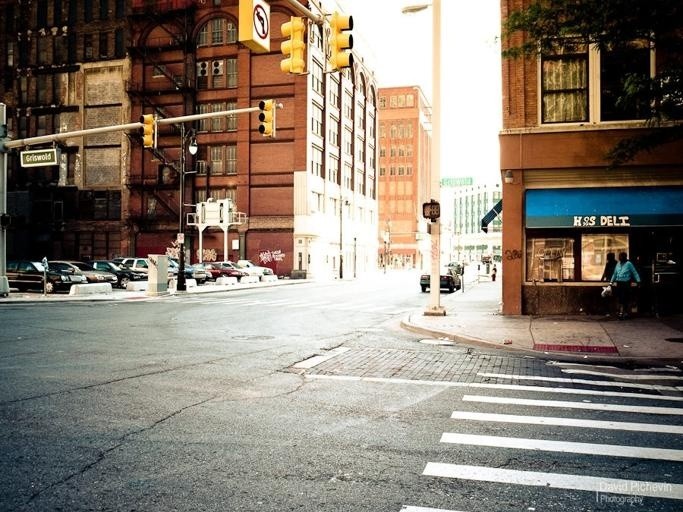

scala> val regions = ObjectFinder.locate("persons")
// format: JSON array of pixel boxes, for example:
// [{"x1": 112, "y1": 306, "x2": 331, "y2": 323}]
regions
[
  {"x1": 607, "y1": 252, "x2": 640, "y2": 319},
  {"x1": 600, "y1": 251, "x2": 618, "y2": 282},
  {"x1": 490, "y1": 264, "x2": 497, "y2": 281}
]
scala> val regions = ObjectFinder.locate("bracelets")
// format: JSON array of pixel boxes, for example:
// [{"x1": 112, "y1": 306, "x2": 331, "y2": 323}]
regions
[{"x1": 609, "y1": 283, "x2": 611, "y2": 285}]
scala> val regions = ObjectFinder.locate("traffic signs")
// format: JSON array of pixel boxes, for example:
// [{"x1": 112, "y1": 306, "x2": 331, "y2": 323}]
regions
[{"x1": 19, "y1": 149, "x2": 58, "y2": 167}]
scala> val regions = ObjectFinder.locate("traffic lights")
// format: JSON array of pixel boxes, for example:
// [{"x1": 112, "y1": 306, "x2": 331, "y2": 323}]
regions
[
  {"x1": 330, "y1": 10, "x2": 354, "y2": 72},
  {"x1": 280, "y1": 15, "x2": 305, "y2": 76},
  {"x1": 138, "y1": 113, "x2": 156, "y2": 149},
  {"x1": 421, "y1": 199, "x2": 440, "y2": 219},
  {"x1": 258, "y1": 99, "x2": 275, "y2": 137}
]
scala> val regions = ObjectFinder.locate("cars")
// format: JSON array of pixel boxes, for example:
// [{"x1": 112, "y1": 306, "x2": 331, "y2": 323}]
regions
[
  {"x1": 418, "y1": 250, "x2": 502, "y2": 293},
  {"x1": 6, "y1": 255, "x2": 275, "y2": 292}
]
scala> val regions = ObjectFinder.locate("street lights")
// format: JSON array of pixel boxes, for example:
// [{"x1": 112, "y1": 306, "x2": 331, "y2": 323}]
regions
[
  {"x1": 337, "y1": 194, "x2": 350, "y2": 279},
  {"x1": 177, "y1": 122, "x2": 199, "y2": 292},
  {"x1": 400, "y1": 0, "x2": 448, "y2": 318}
]
